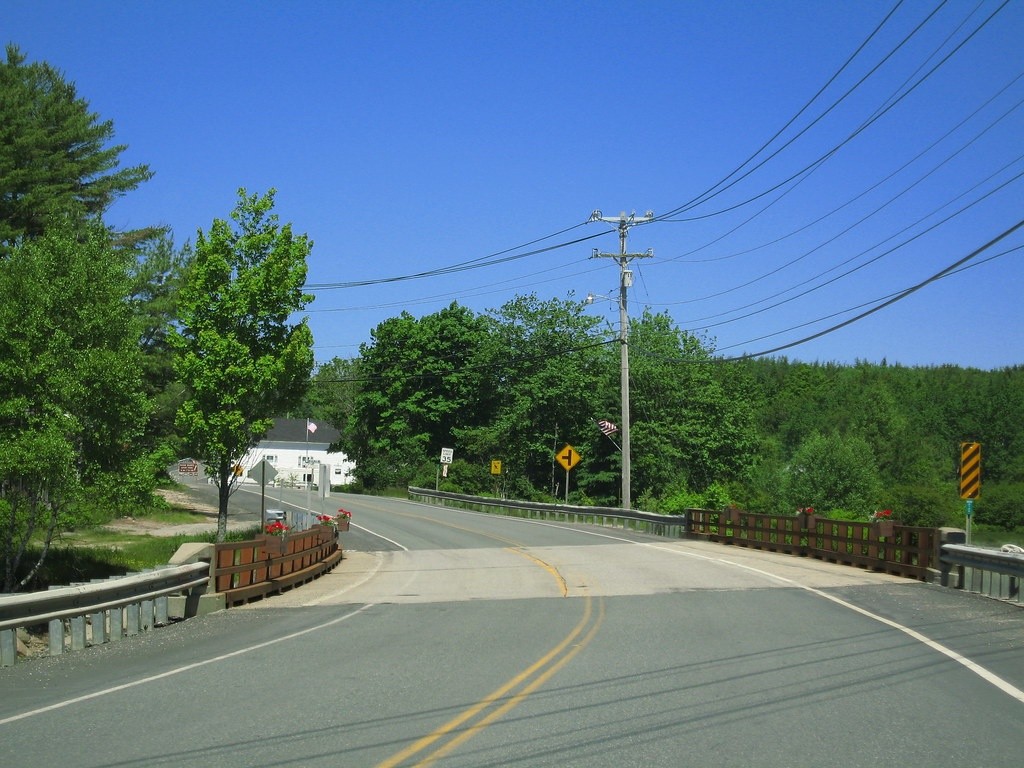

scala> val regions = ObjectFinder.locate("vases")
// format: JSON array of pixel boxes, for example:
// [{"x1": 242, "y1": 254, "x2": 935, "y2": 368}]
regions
[
  {"x1": 254, "y1": 532, "x2": 288, "y2": 555},
  {"x1": 872, "y1": 520, "x2": 902, "y2": 537},
  {"x1": 728, "y1": 510, "x2": 746, "y2": 520},
  {"x1": 798, "y1": 514, "x2": 822, "y2": 529},
  {"x1": 312, "y1": 524, "x2": 336, "y2": 541},
  {"x1": 334, "y1": 518, "x2": 349, "y2": 531}
]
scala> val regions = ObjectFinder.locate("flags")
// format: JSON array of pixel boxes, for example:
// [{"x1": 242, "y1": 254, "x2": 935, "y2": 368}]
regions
[
  {"x1": 598, "y1": 421, "x2": 618, "y2": 436},
  {"x1": 308, "y1": 422, "x2": 318, "y2": 433}
]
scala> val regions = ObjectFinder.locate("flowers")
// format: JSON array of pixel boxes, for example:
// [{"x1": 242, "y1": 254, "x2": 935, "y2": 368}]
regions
[
  {"x1": 264, "y1": 521, "x2": 297, "y2": 537},
  {"x1": 796, "y1": 507, "x2": 816, "y2": 515},
  {"x1": 728, "y1": 504, "x2": 737, "y2": 511},
  {"x1": 336, "y1": 509, "x2": 352, "y2": 522},
  {"x1": 315, "y1": 513, "x2": 336, "y2": 527},
  {"x1": 868, "y1": 511, "x2": 899, "y2": 521}
]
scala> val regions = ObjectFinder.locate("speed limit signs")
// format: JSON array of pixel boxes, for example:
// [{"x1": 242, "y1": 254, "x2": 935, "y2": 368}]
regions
[{"x1": 440, "y1": 448, "x2": 454, "y2": 464}]
